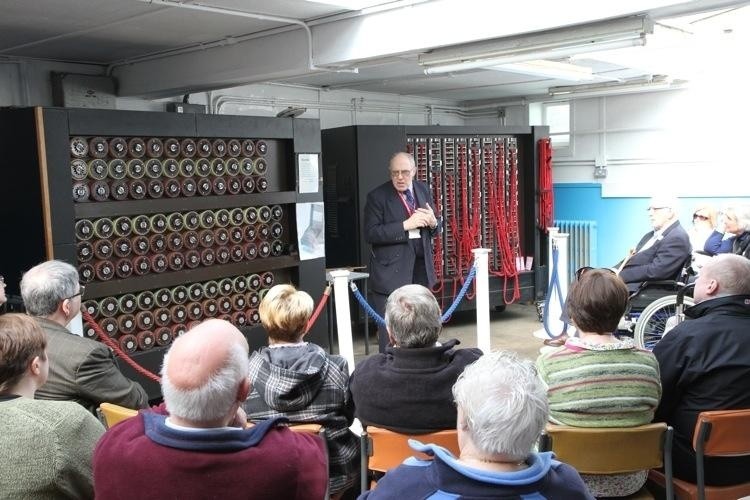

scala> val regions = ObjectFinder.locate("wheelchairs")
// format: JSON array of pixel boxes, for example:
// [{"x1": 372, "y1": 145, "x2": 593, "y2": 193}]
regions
[{"x1": 625, "y1": 254, "x2": 697, "y2": 351}]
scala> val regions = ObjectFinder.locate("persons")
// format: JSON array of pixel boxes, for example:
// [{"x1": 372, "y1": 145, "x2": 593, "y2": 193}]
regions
[
  {"x1": 652, "y1": 255, "x2": 750, "y2": 487},
  {"x1": 704, "y1": 206, "x2": 737, "y2": 255},
  {"x1": 543, "y1": 193, "x2": 692, "y2": 347},
  {"x1": 725, "y1": 203, "x2": 750, "y2": 261},
  {"x1": 690, "y1": 206, "x2": 717, "y2": 273},
  {"x1": 534, "y1": 269, "x2": 662, "y2": 500},
  {"x1": 238, "y1": 285, "x2": 363, "y2": 495},
  {"x1": 0, "y1": 313, "x2": 107, "y2": 500},
  {"x1": 349, "y1": 285, "x2": 484, "y2": 484},
  {"x1": 357, "y1": 348, "x2": 596, "y2": 500},
  {"x1": 0, "y1": 274, "x2": 9, "y2": 306},
  {"x1": 365, "y1": 151, "x2": 442, "y2": 354},
  {"x1": 94, "y1": 318, "x2": 329, "y2": 500},
  {"x1": 20, "y1": 260, "x2": 150, "y2": 420}
]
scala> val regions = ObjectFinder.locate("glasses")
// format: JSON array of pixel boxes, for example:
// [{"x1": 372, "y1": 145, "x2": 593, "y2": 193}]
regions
[
  {"x1": 647, "y1": 206, "x2": 664, "y2": 211},
  {"x1": 57, "y1": 284, "x2": 87, "y2": 302},
  {"x1": 693, "y1": 213, "x2": 710, "y2": 222},
  {"x1": 392, "y1": 170, "x2": 410, "y2": 177}
]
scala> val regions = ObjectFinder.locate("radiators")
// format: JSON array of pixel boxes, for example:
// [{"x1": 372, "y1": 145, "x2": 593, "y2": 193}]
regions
[{"x1": 553, "y1": 219, "x2": 598, "y2": 285}]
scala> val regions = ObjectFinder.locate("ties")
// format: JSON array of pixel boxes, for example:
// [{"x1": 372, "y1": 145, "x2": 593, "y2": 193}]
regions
[{"x1": 403, "y1": 189, "x2": 416, "y2": 215}]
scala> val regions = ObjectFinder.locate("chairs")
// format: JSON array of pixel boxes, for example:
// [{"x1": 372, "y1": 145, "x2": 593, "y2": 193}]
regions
[
  {"x1": 246, "y1": 422, "x2": 329, "y2": 500},
  {"x1": 361, "y1": 425, "x2": 461, "y2": 500},
  {"x1": 96, "y1": 404, "x2": 141, "y2": 430},
  {"x1": 649, "y1": 409, "x2": 750, "y2": 500},
  {"x1": 539, "y1": 422, "x2": 675, "y2": 500}
]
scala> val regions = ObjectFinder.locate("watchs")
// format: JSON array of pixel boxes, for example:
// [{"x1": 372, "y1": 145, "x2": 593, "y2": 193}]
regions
[{"x1": 431, "y1": 222, "x2": 438, "y2": 229}]
